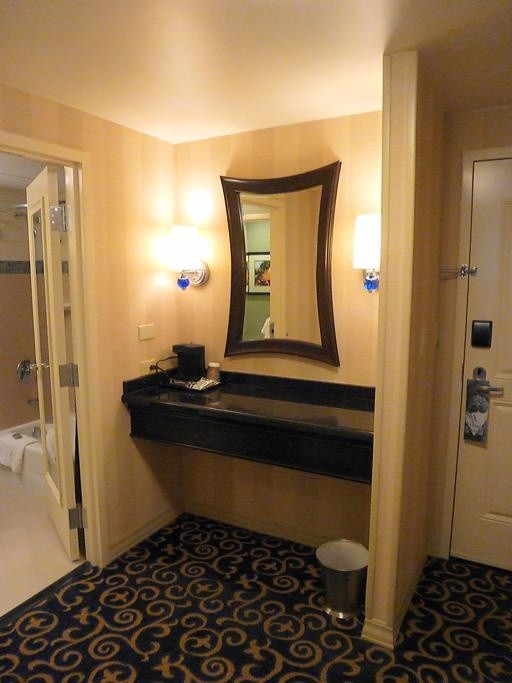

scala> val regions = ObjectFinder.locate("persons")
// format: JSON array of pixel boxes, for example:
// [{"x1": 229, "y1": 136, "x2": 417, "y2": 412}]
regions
[{"x1": 255, "y1": 261, "x2": 270, "y2": 286}]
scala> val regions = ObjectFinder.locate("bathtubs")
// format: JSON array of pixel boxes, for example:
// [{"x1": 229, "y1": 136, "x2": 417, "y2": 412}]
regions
[{"x1": 2, "y1": 418, "x2": 59, "y2": 483}]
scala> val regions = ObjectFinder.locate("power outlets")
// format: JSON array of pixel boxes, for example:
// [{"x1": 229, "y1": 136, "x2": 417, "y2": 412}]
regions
[{"x1": 139, "y1": 359, "x2": 158, "y2": 376}]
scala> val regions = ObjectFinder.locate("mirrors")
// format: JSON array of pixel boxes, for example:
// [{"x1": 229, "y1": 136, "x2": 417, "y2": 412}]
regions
[{"x1": 220, "y1": 160, "x2": 341, "y2": 369}]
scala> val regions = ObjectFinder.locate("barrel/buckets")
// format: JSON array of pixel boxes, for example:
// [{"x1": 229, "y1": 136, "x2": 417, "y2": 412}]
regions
[{"x1": 316, "y1": 539, "x2": 369, "y2": 607}]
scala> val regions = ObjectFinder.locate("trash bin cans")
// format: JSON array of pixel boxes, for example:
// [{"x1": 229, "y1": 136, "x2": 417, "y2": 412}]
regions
[{"x1": 315, "y1": 538, "x2": 368, "y2": 619}]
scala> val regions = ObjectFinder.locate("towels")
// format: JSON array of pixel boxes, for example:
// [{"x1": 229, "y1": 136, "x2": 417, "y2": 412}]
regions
[{"x1": 0, "y1": 432, "x2": 39, "y2": 475}]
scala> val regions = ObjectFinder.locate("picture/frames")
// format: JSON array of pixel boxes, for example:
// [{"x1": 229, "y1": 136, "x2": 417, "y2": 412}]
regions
[{"x1": 245, "y1": 252, "x2": 271, "y2": 295}]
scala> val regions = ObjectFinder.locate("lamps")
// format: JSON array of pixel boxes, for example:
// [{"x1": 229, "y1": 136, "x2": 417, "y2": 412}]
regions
[{"x1": 350, "y1": 213, "x2": 382, "y2": 291}]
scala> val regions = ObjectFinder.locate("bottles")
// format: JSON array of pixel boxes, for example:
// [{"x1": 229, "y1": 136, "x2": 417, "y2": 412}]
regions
[{"x1": 206, "y1": 362, "x2": 220, "y2": 383}]
